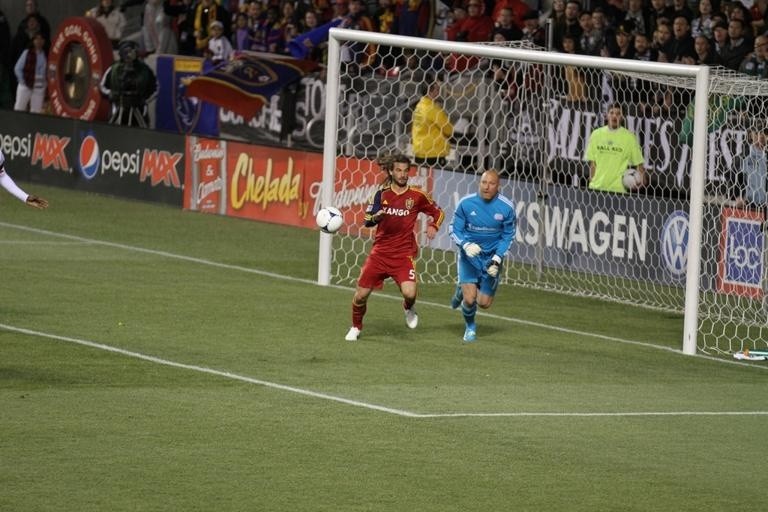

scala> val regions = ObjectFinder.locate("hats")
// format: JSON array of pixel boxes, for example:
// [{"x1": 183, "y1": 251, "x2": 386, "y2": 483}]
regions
[
  {"x1": 710, "y1": 11, "x2": 727, "y2": 22},
  {"x1": 210, "y1": 21, "x2": 223, "y2": 29},
  {"x1": 521, "y1": 10, "x2": 540, "y2": 20},
  {"x1": 614, "y1": 24, "x2": 630, "y2": 36}
]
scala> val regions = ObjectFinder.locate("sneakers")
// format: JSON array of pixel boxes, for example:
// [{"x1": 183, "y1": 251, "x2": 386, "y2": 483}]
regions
[
  {"x1": 402, "y1": 302, "x2": 418, "y2": 329},
  {"x1": 463, "y1": 323, "x2": 477, "y2": 342},
  {"x1": 452, "y1": 282, "x2": 463, "y2": 308},
  {"x1": 345, "y1": 326, "x2": 361, "y2": 341}
]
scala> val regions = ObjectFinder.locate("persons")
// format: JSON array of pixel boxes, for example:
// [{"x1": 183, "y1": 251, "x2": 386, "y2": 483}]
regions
[
  {"x1": 86, "y1": 1, "x2": 767, "y2": 208},
  {"x1": 1, "y1": 1, "x2": 51, "y2": 113},
  {"x1": 345, "y1": 155, "x2": 444, "y2": 341},
  {"x1": 449, "y1": 169, "x2": 516, "y2": 342},
  {"x1": 0, "y1": 153, "x2": 48, "y2": 208}
]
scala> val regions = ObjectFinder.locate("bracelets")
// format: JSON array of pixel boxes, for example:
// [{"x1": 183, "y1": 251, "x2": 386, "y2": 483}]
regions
[
  {"x1": 463, "y1": 242, "x2": 470, "y2": 249},
  {"x1": 492, "y1": 254, "x2": 502, "y2": 264}
]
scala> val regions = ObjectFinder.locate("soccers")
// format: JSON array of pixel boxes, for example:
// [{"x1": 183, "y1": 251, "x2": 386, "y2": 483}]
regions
[{"x1": 317, "y1": 207, "x2": 341, "y2": 234}]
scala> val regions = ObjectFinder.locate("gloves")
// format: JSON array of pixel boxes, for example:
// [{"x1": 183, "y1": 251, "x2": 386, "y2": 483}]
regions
[
  {"x1": 462, "y1": 242, "x2": 482, "y2": 258},
  {"x1": 486, "y1": 255, "x2": 502, "y2": 278}
]
